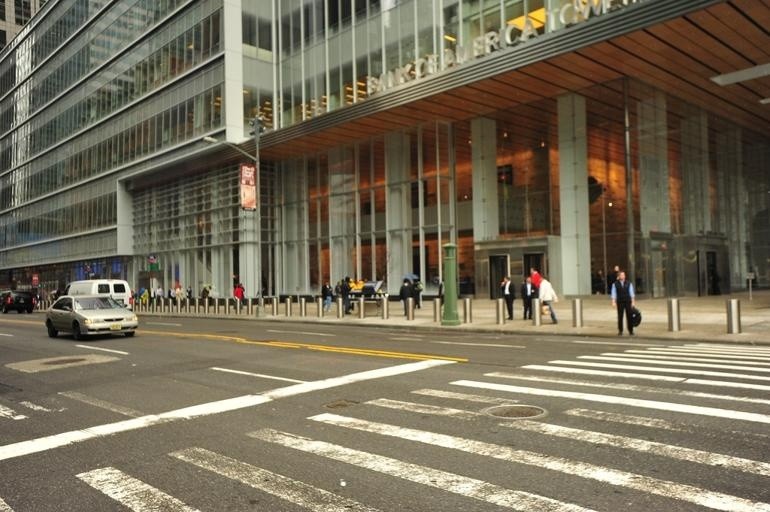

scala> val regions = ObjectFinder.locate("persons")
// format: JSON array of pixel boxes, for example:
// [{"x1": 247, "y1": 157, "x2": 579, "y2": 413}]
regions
[
  {"x1": 710, "y1": 264, "x2": 722, "y2": 296},
  {"x1": 607, "y1": 265, "x2": 620, "y2": 294},
  {"x1": 321, "y1": 275, "x2": 423, "y2": 315},
  {"x1": 529, "y1": 265, "x2": 542, "y2": 298},
  {"x1": 434, "y1": 276, "x2": 444, "y2": 307},
  {"x1": 520, "y1": 276, "x2": 536, "y2": 319},
  {"x1": 34, "y1": 280, "x2": 246, "y2": 311},
  {"x1": 610, "y1": 271, "x2": 636, "y2": 336},
  {"x1": 538, "y1": 276, "x2": 560, "y2": 324},
  {"x1": 592, "y1": 269, "x2": 605, "y2": 295},
  {"x1": 499, "y1": 276, "x2": 516, "y2": 320}
]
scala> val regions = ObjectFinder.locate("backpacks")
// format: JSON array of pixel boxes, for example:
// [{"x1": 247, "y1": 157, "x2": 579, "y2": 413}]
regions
[{"x1": 630, "y1": 306, "x2": 642, "y2": 328}]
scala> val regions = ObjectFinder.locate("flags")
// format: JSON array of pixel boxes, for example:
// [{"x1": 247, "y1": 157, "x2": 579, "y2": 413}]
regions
[{"x1": 239, "y1": 162, "x2": 257, "y2": 211}]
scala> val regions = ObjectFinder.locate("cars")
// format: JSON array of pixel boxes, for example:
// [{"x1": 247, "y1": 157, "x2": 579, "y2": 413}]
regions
[
  {"x1": 45, "y1": 295, "x2": 139, "y2": 341},
  {"x1": 0, "y1": 290, "x2": 36, "y2": 314}
]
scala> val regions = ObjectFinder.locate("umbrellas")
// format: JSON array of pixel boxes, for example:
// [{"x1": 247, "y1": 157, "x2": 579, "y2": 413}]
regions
[{"x1": 401, "y1": 272, "x2": 418, "y2": 280}]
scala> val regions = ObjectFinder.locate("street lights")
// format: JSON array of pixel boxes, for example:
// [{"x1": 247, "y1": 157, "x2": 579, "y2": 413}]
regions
[{"x1": 202, "y1": 115, "x2": 266, "y2": 297}]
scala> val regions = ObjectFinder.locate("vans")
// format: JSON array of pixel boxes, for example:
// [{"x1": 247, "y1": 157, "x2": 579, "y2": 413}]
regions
[{"x1": 63, "y1": 279, "x2": 133, "y2": 312}]
